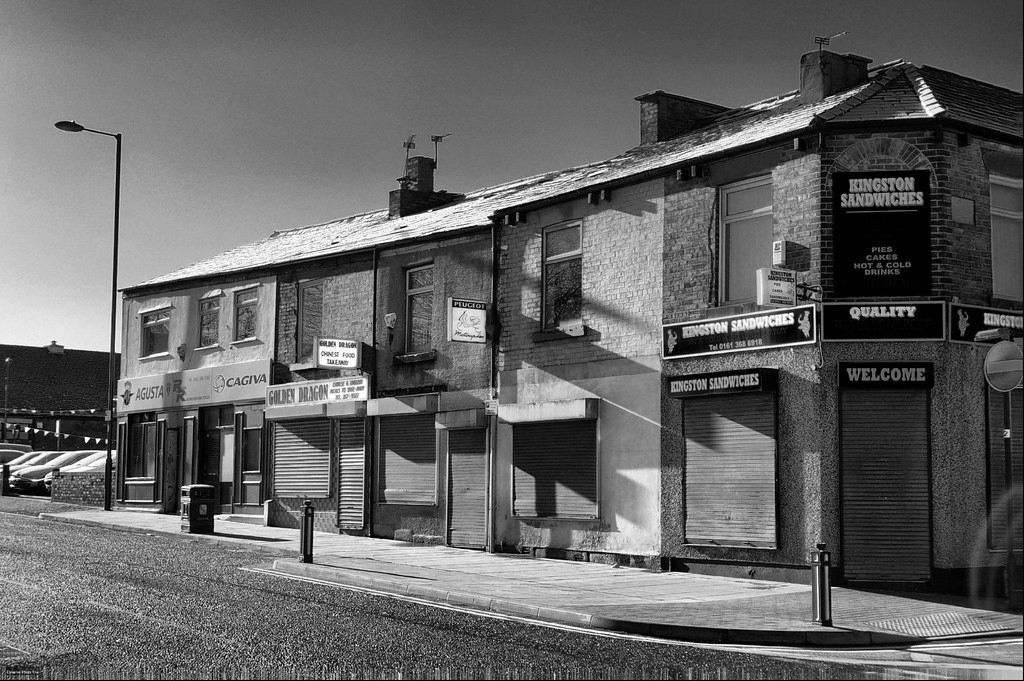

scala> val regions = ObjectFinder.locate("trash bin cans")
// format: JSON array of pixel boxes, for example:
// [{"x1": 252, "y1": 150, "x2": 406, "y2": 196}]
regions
[{"x1": 179, "y1": 484, "x2": 216, "y2": 534}]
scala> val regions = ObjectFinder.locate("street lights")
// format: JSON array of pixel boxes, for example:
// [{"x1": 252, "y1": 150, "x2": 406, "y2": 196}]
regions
[{"x1": 53, "y1": 119, "x2": 122, "y2": 512}]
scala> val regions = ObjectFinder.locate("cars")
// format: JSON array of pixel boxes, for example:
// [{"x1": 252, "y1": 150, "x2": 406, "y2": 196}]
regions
[
  {"x1": 44, "y1": 449, "x2": 117, "y2": 496},
  {"x1": 10, "y1": 450, "x2": 105, "y2": 496},
  {"x1": 0, "y1": 452, "x2": 42, "y2": 489},
  {"x1": 0, "y1": 451, "x2": 67, "y2": 496}
]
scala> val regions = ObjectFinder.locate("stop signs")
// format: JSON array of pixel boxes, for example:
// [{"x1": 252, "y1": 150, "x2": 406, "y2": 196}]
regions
[{"x1": 985, "y1": 339, "x2": 1024, "y2": 394}]
